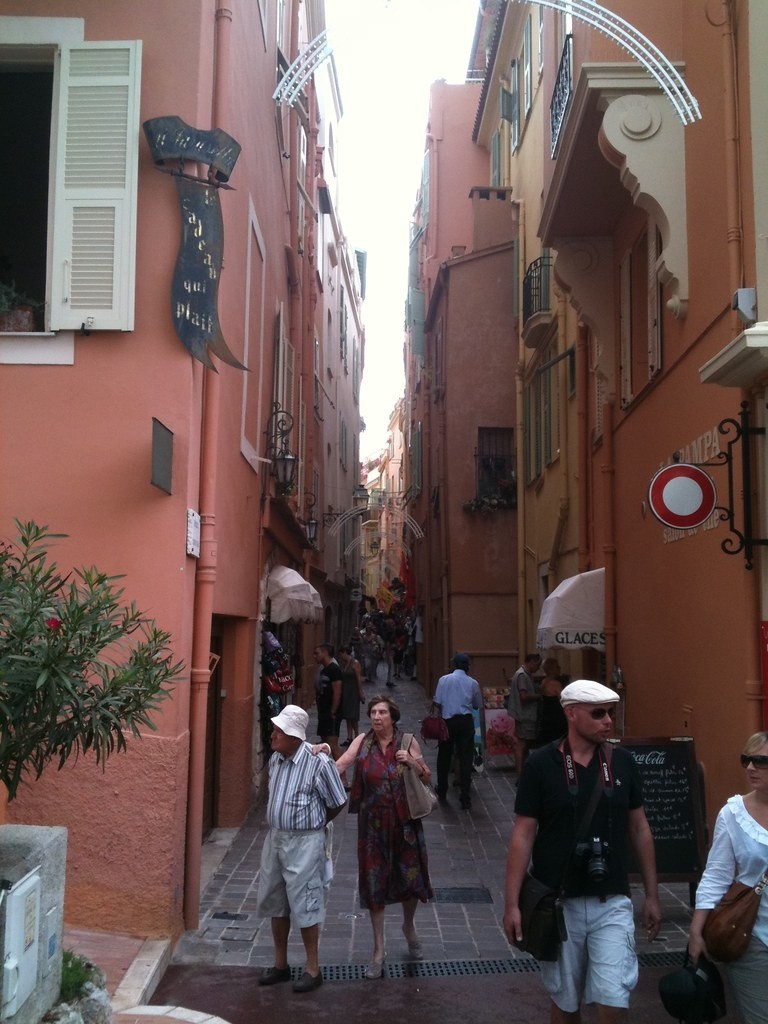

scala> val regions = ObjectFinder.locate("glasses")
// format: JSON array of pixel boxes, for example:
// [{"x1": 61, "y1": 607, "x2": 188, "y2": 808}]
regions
[
  {"x1": 571, "y1": 705, "x2": 615, "y2": 719},
  {"x1": 740, "y1": 754, "x2": 768, "y2": 769}
]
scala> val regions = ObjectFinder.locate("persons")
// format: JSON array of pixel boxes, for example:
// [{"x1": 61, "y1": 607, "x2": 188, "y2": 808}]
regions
[
  {"x1": 256, "y1": 705, "x2": 348, "y2": 991},
  {"x1": 508, "y1": 654, "x2": 564, "y2": 785},
  {"x1": 312, "y1": 642, "x2": 366, "y2": 791},
  {"x1": 312, "y1": 696, "x2": 433, "y2": 977},
  {"x1": 503, "y1": 680, "x2": 662, "y2": 1024},
  {"x1": 347, "y1": 608, "x2": 418, "y2": 685},
  {"x1": 688, "y1": 731, "x2": 768, "y2": 1024},
  {"x1": 435, "y1": 654, "x2": 483, "y2": 809}
]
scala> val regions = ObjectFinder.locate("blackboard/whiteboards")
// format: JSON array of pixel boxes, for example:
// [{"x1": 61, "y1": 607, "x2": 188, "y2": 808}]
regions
[{"x1": 606, "y1": 736, "x2": 706, "y2": 882}]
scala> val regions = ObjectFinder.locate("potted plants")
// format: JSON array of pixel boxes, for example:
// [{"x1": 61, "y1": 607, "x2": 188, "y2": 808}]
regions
[{"x1": 0, "y1": 279, "x2": 47, "y2": 332}]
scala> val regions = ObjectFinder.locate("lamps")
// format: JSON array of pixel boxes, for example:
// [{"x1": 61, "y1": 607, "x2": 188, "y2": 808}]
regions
[
  {"x1": 304, "y1": 488, "x2": 318, "y2": 540},
  {"x1": 367, "y1": 542, "x2": 379, "y2": 560},
  {"x1": 265, "y1": 401, "x2": 296, "y2": 495},
  {"x1": 355, "y1": 484, "x2": 370, "y2": 515}
]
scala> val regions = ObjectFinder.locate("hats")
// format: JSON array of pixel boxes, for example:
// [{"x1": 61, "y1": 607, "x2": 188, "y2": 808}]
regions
[
  {"x1": 560, "y1": 679, "x2": 619, "y2": 708},
  {"x1": 270, "y1": 704, "x2": 309, "y2": 741}
]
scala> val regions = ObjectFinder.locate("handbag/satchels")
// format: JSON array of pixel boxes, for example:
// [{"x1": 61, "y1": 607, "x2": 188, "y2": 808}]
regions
[
  {"x1": 422, "y1": 705, "x2": 449, "y2": 741},
  {"x1": 702, "y1": 868, "x2": 768, "y2": 961},
  {"x1": 659, "y1": 955, "x2": 725, "y2": 1023},
  {"x1": 400, "y1": 733, "x2": 438, "y2": 819},
  {"x1": 512, "y1": 872, "x2": 562, "y2": 960}
]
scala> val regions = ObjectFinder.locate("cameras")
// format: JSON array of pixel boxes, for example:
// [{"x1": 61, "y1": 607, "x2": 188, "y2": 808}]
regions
[{"x1": 576, "y1": 836, "x2": 611, "y2": 882}]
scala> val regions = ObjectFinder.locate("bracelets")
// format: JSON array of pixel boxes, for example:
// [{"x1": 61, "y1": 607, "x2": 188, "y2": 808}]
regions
[
  {"x1": 419, "y1": 766, "x2": 426, "y2": 777},
  {"x1": 324, "y1": 743, "x2": 331, "y2": 756}
]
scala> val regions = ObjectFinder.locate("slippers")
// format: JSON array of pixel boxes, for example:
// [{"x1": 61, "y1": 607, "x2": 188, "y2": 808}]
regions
[
  {"x1": 408, "y1": 936, "x2": 423, "y2": 958},
  {"x1": 366, "y1": 951, "x2": 387, "y2": 979}
]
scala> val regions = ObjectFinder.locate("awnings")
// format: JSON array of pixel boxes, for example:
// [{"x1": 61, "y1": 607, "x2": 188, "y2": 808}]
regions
[
  {"x1": 268, "y1": 565, "x2": 323, "y2": 624},
  {"x1": 535, "y1": 567, "x2": 605, "y2": 653}
]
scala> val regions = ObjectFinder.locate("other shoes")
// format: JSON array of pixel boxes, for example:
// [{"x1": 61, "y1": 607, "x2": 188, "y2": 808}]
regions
[
  {"x1": 435, "y1": 785, "x2": 445, "y2": 800},
  {"x1": 293, "y1": 971, "x2": 322, "y2": 992},
  {"x1": 340, "y1": 738, "x2": 353, "y2": 746},
  {"x1": 459, "y1": 793, "x2": 471, "y2": 809},
  {"x1": 386, "y1": 682, "x2": 395, "y2": 686},
  {"x1": 261, "y1": 966, "x2": 291, "y2": 984}
]
